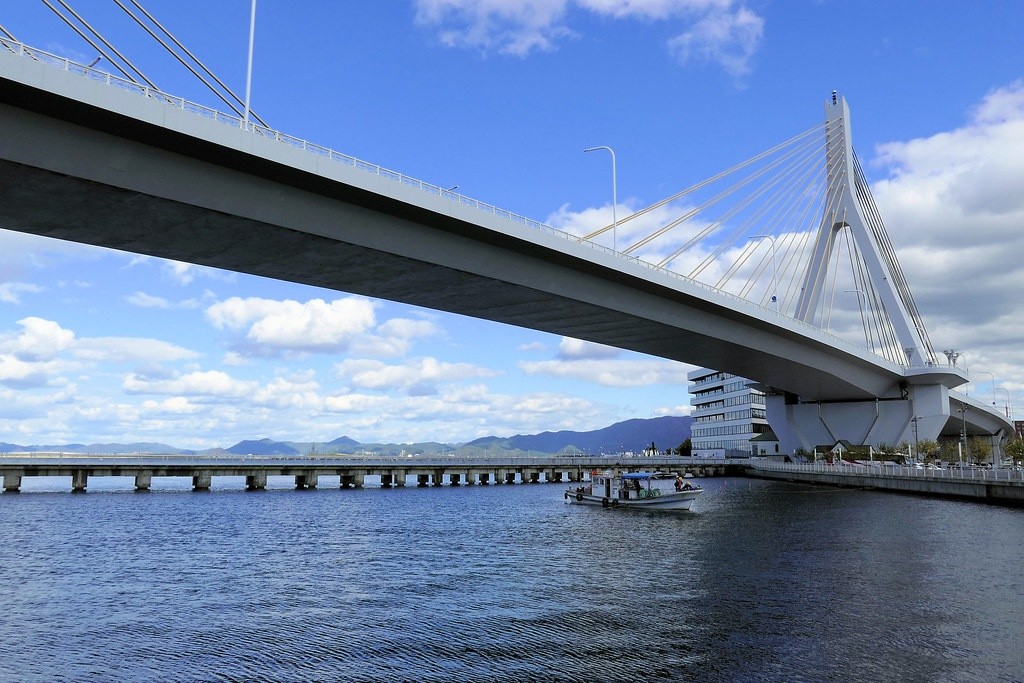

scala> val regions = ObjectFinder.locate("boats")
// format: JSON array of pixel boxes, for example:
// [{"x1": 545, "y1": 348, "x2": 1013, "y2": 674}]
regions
[{"x1": 564, "y1": 472, "x2": 705, "y2": 510}]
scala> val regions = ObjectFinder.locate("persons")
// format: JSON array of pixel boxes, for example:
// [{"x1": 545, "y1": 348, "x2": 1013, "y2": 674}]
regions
[
  {"x1": 674, "y1": 476, "x2": 683, "y2": 491},
  {"x1": 683, "y1": 481, "x2": 693, "y2": 491}
]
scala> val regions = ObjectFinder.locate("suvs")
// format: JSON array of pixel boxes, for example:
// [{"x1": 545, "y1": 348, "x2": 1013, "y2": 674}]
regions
[
  {"x1": 912, "y1": 463, "x2": 946, "y2": 471},
  {"x1": 948, "y1": 462, "x2": 992, "y2": 470}
]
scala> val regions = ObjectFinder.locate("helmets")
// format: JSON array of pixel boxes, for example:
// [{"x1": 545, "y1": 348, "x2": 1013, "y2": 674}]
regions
[
  {"x1": 678, "y1": 477, "x2": 682, "y2": 482},
  {"x1": 686, "y1": 481, "x2": 691, "y2": 485}
]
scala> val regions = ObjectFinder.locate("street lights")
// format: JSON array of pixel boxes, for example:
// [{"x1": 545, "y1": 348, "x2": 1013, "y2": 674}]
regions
[
  {"x1": 911, "y1": 414, "x2": 922, "y2": 463},
  {"x1": 941, "y1": 348, "x2": 1016, "y2": 420},
  {"x1": 843, "y1": 290, "x2": 870, "y2": 351},
  {"x1": 957, "y1": 403, "x2": 968, "y2": 461},
  {"x1": 583, "y1": 145, "x2": 620, "y2": 259},
  {"x1": 748, "y1": 235, "x2": 779, "y2": 311}
]
